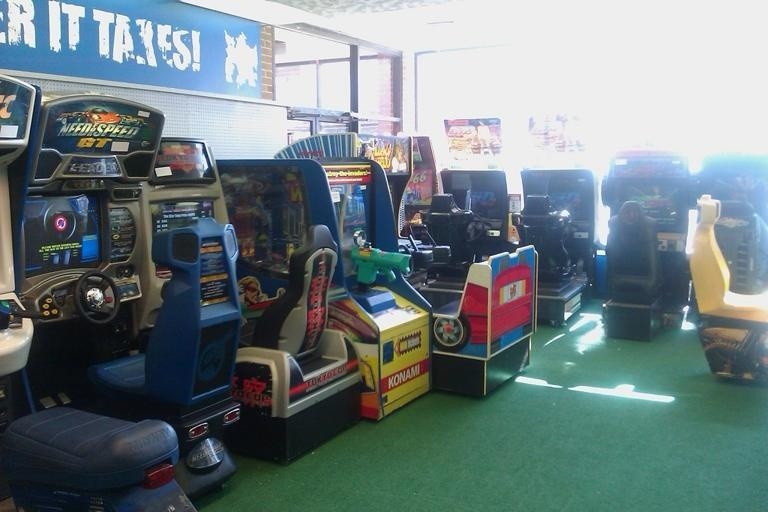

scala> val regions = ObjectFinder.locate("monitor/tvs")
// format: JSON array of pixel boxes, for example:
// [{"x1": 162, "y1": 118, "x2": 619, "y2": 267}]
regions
[
  {"x1": 151, "y1": 201, "x2": 213, "y2": 278},
  {"x1": 471, "y1": 191, "x2": 500, "y2": 218},
  {"x1": 549, "y1": 192, "x2": 586, "y2": 220},
  {"x1": 625, "y1": 184, "x2": 680, "y2": 230},
  {"x1": 722, "y1": 175, "x2": 759, "y2": 200},
  {"x1": 329, "y1": 183, "x2": 370, "y2": 277},
  {"x1": 24, "y1": 195, "x2": 102, "y2": 278}
]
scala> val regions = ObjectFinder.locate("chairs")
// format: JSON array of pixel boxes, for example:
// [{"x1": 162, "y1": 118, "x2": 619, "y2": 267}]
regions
[
  {"x1": 429, "y1": 243, "x2": 539, "y2": 400},
  {"x1": 685, "y1": 194, "x2": 767, "y2": 327},
  {"x1": 86, "y1": 220, "x2": 245, "y2": 418},
  {"x1": 415, "y1": 190, "x2": 490, "y2": 278},
  {"x1": 234, "y1": 224, "x2": 337, "y2": 361},
  {"x1": 512, "y1": 192, "x2": 580, "y2": 282},
  {"x1": 599, "y1": 197, "x2": 657, "y2": 295}
]
